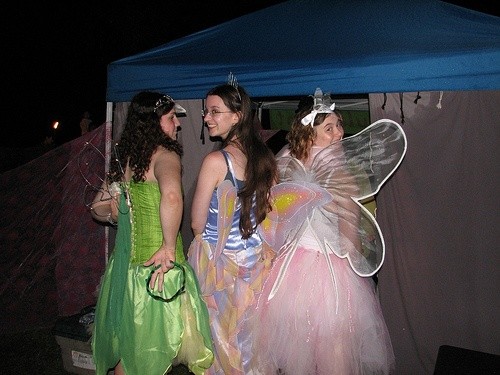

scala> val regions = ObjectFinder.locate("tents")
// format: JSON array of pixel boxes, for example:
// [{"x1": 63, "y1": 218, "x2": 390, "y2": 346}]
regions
[{"x1": 105, "y1": 1, "x2": 499, "y2": 375}]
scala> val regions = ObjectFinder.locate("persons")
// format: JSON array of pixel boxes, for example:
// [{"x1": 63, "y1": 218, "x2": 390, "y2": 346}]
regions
[
  {"x1": 253, "y1": 87, "x2": 395, "y2": 375},
  {"x1": 91, "y1": 90, "x2": 215, "y2": 375},
  {"x1": 188, "y1": 72, "x2": 281, "y2": 375}
]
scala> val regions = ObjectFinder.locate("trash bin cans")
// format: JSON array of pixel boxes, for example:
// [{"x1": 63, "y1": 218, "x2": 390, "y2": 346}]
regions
[{"x1": 51, "y1": 304, "x2": 114, "y2": 375}]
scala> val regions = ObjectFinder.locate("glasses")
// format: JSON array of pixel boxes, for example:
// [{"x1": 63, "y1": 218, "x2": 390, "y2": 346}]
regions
[{"x1": 201, "y1": 109, "x2": 234, "y2": 116}]
